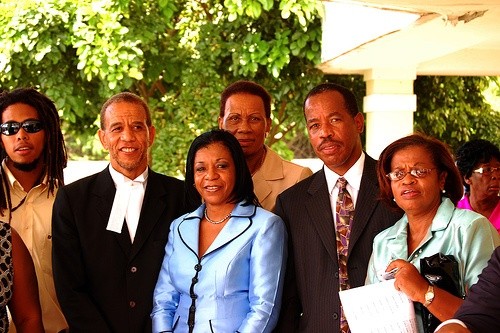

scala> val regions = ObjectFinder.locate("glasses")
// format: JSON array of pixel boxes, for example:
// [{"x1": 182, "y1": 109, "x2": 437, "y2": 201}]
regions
[
  {"x1": 473, "y1": 166, "x2": 500, "y2": 176},
  {"x1": 0, "y1": 118, "x2": 44, "y2": 136},
  {"x1": 386, "y1": 167, "x2": 436, "y2": 181}
]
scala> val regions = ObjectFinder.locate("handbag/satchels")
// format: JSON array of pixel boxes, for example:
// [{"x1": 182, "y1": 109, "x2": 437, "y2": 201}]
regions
[{"x1": 420, "y1": 252, "x2": 464, "y2": 333}]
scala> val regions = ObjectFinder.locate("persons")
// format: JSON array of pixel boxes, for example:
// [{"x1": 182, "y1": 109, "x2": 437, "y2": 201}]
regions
[
  {"x1": 0, "y1": 162, "x2": 45, "y2": 333},
  {"x1": 218, "y1": 81, "x2": 313, "y2": 215},
  {"x1": 364, "y1": 134, "x2": 500, "y2": 333},
  {"x1": 434, "y1": 245, "x2": 500, "y2": 333},
  {"x1": 275, "y1": 83, "x2": 406, "y2": 333},
  {"x1": 52, "y1": 93, "x2": 202, "y2": 333},
  {"x1": 456, "y1": 139, "x2": 500, "y2": 235},
  {"x1": 150, "y1": 129, "x2": 288, "y2": 333},
  {"x1": 0, "y1": 88, "x2": 71, "y2": 333}
]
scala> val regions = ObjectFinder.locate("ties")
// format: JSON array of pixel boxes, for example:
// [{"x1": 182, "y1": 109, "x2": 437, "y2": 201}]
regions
[
  {"x1": 335, "y1": 176, "x2": 356, "y2": 333},
  {"x1": 105, "y1": 180, "x2": 145, "y2": 244}
]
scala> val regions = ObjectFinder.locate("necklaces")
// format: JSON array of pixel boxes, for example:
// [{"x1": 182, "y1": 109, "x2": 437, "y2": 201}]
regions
[
  {"x1": 204, "y1": 208, "x2": 232, "y2": 224},
  {"x1": 1, "y1": 164, "x2": 46, "y2": 224}
]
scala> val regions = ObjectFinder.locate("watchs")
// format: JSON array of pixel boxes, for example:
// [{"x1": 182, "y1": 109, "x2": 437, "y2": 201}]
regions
[{"x1": 423, "y1": 285, "x2": 434, "y2": 307}]
